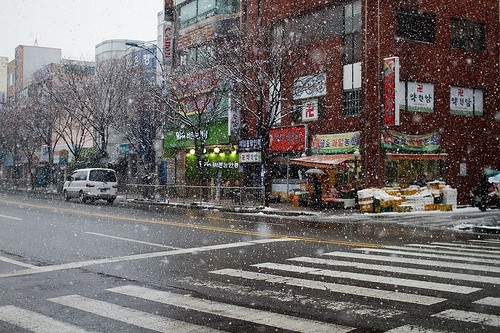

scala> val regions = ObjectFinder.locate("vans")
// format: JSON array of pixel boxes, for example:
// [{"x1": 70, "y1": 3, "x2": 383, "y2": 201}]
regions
[{"x1": 62, "y1": 168, "x2": 118, "y2": 203}]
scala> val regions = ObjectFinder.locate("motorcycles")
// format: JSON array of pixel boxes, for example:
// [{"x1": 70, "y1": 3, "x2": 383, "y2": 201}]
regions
[{"x1": 476, "y1": 184, "x2": 500, "y2": 211}]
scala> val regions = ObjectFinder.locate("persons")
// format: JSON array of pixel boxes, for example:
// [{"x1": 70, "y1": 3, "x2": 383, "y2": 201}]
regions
[{"x1": 310, "y1": 174, "x2": 329, "y2": 212}]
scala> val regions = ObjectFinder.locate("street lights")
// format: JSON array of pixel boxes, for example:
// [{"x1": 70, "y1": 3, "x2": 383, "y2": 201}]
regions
[{"x1": 126, "y1": 42, "x2": 165, "y2": 201}]
